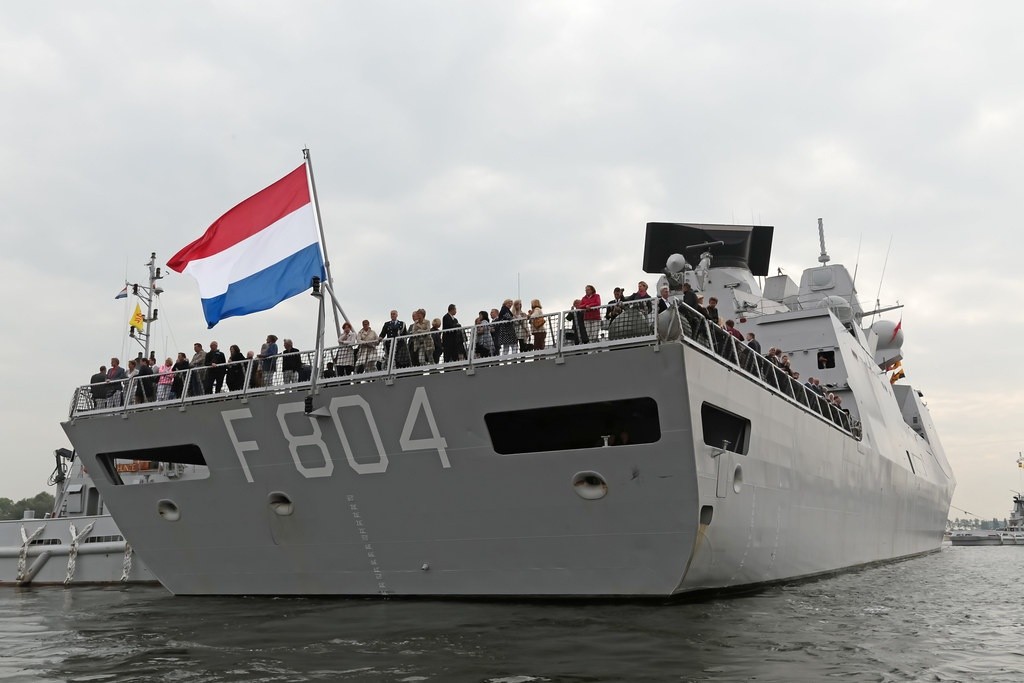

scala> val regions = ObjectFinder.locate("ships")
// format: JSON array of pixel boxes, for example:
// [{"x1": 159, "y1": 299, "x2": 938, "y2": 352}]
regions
[{"x1": 2, "y1": 218, "x2": 957, "y2": 599}]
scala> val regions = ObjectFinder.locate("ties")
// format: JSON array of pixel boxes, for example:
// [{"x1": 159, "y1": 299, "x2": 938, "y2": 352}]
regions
[{"x1": 393, "y1": 321, "x2": 396, "y2": 326}]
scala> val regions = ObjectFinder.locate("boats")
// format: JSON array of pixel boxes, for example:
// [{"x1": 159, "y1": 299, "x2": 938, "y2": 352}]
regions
[{"x1": 951, "y1": 489, "x2": 1024, "y2": 547}]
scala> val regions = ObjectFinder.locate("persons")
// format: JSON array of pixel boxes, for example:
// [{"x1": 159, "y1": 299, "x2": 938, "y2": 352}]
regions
[{"x1": 88, "y1": 282, "x2": 861, "y2": 439}]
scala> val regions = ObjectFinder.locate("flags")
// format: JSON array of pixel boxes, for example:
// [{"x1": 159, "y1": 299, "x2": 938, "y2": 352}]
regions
[{"x1": 166, "y1": 165, "x2": 327, "y2": 329}]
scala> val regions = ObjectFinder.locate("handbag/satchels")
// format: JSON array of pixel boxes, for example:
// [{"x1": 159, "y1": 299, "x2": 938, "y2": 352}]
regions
[{"x1": 531, "y1": 316, "x2": 547, "y2": 329}]
